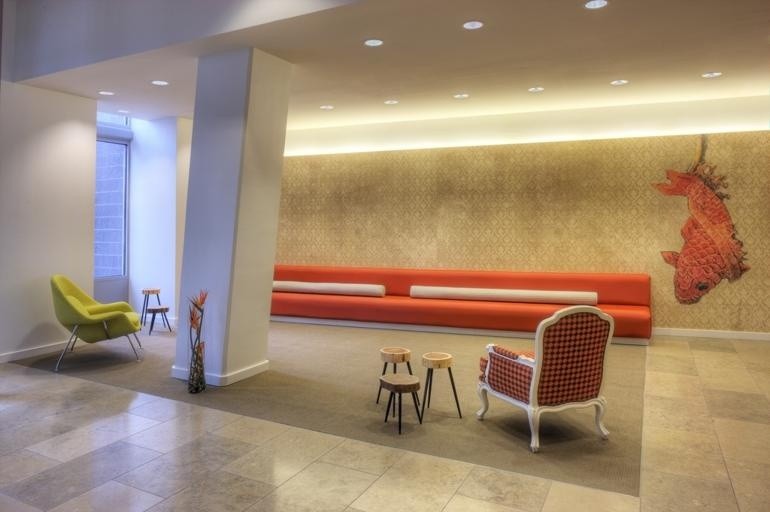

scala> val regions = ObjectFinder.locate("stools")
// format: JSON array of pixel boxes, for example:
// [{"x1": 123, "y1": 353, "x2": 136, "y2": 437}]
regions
[
  {"x1": 375, "y1": 344, "x2": 421, "y2": 417},
  {"x1": 378, "y1": 371, "x2": 423, "y2": 435},
  {"x1": 144, "y1": 305, "x2": 172, "y2": 336},
  {"x1": 420, "y1": 348, "x2": 463, "y2": 421},
  {"x1": 139, "y1": 288, "x2": 168, "y2": 331}
]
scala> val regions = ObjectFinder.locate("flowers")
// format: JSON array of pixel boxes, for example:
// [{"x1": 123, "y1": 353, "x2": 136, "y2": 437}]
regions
[{"x1": 186, "y1": 289, "x2": 209, "y2": 351}]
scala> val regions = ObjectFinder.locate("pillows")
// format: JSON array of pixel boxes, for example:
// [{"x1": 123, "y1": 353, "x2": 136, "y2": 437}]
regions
[
  {"x1": 272, "y1": 279, "x2": 387, "y2": 299},
  {"x1": 409, "y1": 284, "x2": 599, "y2": 307},
  {"x1": 65, "y1": 295, "x2": 90, "y2": 317}
]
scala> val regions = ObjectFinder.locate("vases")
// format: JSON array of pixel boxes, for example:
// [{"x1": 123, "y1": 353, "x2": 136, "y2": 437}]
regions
[{"x1": 185, "y1": 345, "x2": 208, "y2": 396}]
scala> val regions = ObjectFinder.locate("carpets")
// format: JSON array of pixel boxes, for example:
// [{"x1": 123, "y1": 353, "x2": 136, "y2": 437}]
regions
[{"x1": 7, "y1": 317, "x2": 648, "y2": 501}]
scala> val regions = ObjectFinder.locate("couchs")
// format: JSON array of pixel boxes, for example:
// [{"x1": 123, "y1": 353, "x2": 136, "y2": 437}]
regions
[
  {"x1": 270, "y1": 262, "x2": 653, "y2": 348},
  {"x1": 48, "y1": 271, "x2": 145, "y2": 373},
  {"x1": 473, "y1": 303, "x2": 618, "y2": 453}
]
